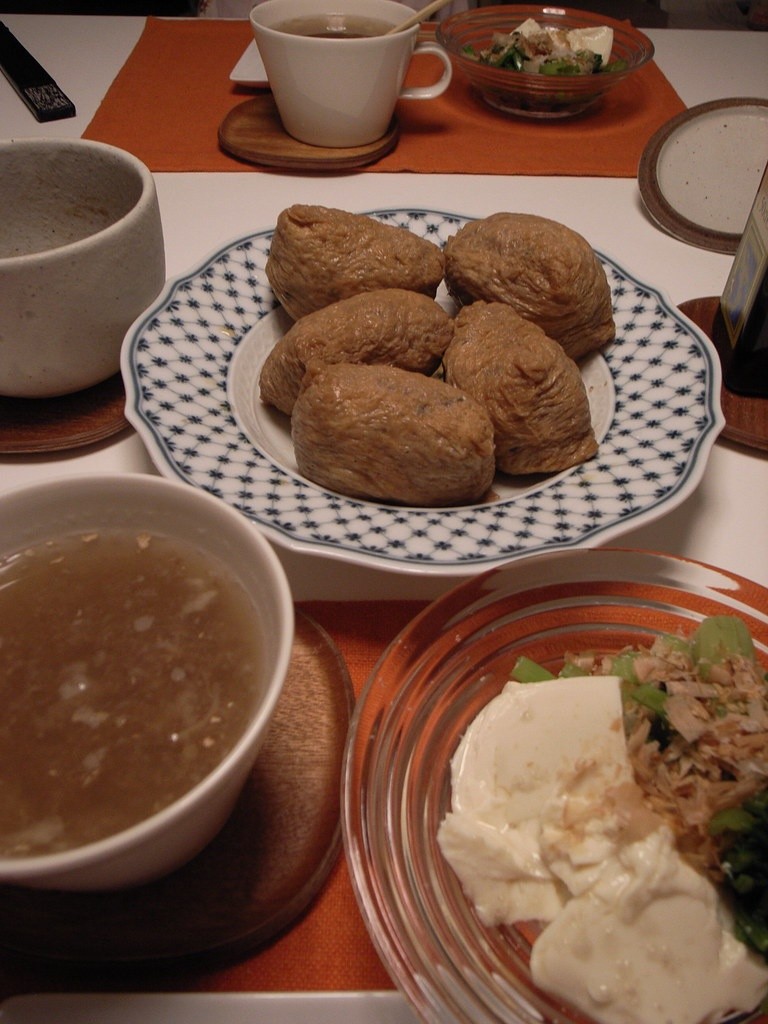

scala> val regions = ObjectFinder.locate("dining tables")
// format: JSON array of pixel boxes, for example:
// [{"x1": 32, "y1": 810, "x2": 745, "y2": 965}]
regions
[{"x1": 0, "y1": 14, "x2": 768, "y2": 1024}]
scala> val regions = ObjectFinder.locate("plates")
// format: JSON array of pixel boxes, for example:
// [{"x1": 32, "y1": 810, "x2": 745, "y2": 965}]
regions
[
  {"x1": 341, "y1": 546, "x2": 768, "y2": 1024},
  {"x1": 118, "y1": 210, "x2": 726, "y2": 576}
]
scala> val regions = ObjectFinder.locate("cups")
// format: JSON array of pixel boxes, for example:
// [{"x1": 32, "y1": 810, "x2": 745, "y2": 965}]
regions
[
  {"x1": 250, "y1": 0, "x2": 452, "y2": 148},
  {"x1": 229, "y1": 36, "x2": 271, "y2": 88}
]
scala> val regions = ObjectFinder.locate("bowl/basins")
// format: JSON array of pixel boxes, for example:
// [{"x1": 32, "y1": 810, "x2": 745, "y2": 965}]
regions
[
  {"x1": 0, "y1": 138, "x2": 165, "y2": 395},
  {"x1": 437, "y1": 4, "x2": 656, "y2": 118},
  {"x1": 0, "y1": 473, "x2": 296, "y2": 887}
]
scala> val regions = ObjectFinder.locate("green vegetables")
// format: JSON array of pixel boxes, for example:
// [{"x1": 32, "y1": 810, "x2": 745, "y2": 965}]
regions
[
  {"x1": 506, "y1": 614, "x2": 768, "y2": 899},
  {"x1": 462, "y1": 43, "x2": 628, "y2": 98}
]
